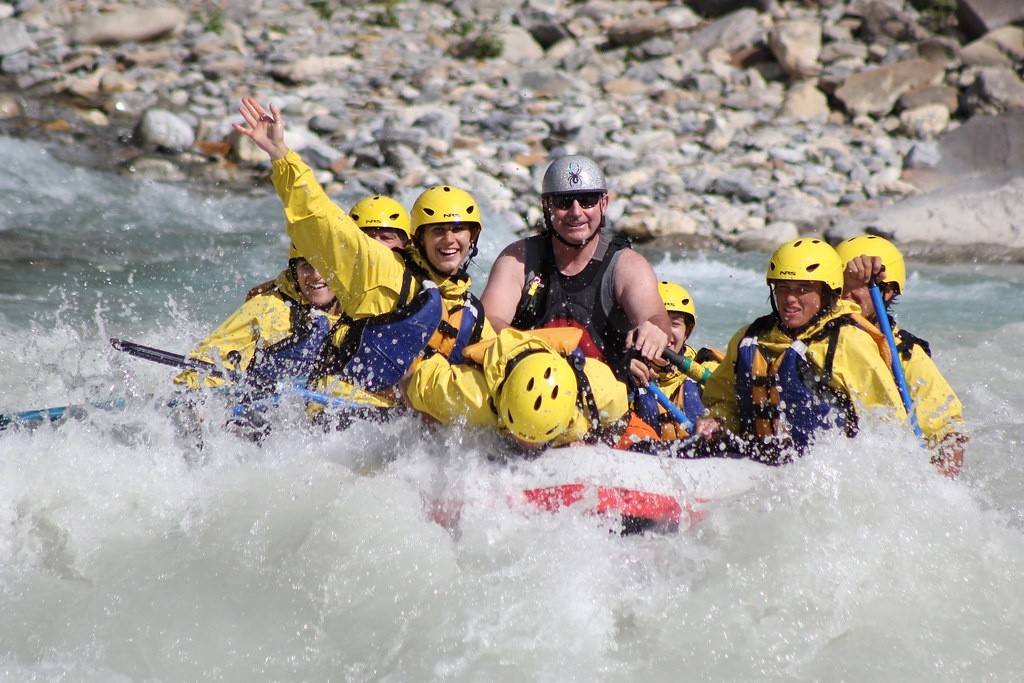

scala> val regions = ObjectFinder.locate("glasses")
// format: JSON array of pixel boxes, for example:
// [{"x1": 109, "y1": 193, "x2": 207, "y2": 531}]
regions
[{"x1": 548, "y1": 193, "x2": 606, "y2": 209}]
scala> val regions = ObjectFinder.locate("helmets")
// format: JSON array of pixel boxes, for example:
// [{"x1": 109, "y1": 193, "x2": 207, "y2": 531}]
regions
[
  {"x1": 766, "y1": 237, "x2": 843, "y2": 299},
  {"x1": 349, "y1": 195, "x2": 413, "y2": 245},
  {"x1": 657, "y1": 280, "x2": 697, "y2": 339},
  {"x1": 835, "y1": 234, "x2": 906, "y2": 295},
  {"x1": 499, "y1": 352, "x2": 577, "y2": 444},
  {"x1": 541, "y1": 153, "x2": 608, "y2": 197},
  {"x1": 288, "y1": 239, "x2": 304, "y2": 268},
  {"x1": 410, "y1": 186, "x2": 482, "y2": 257}
]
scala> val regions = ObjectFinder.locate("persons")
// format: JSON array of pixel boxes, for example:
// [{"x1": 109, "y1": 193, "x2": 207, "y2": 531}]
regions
[{"x1": 175, "y1": 96, "x2": 967, "y2": 479}]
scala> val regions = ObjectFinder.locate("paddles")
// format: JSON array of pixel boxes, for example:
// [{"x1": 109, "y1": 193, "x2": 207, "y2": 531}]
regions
[
  {"x1": 634, "y1": 359, "x2": 697, "y2": 437},
  {"x1": 861, "y1": 264, "x2": 931, "y2": 452},
  {"x1": 109, "y1": 337, "x2": 384, "y2": 419},
  {"x1": 631, "y1": 327, "x2": 714, "y2": 387}
]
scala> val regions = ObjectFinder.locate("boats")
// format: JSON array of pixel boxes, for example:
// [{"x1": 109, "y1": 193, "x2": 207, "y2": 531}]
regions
[{"x1": 422, "y1": 444, "x2": 766, "y2": 537}]
